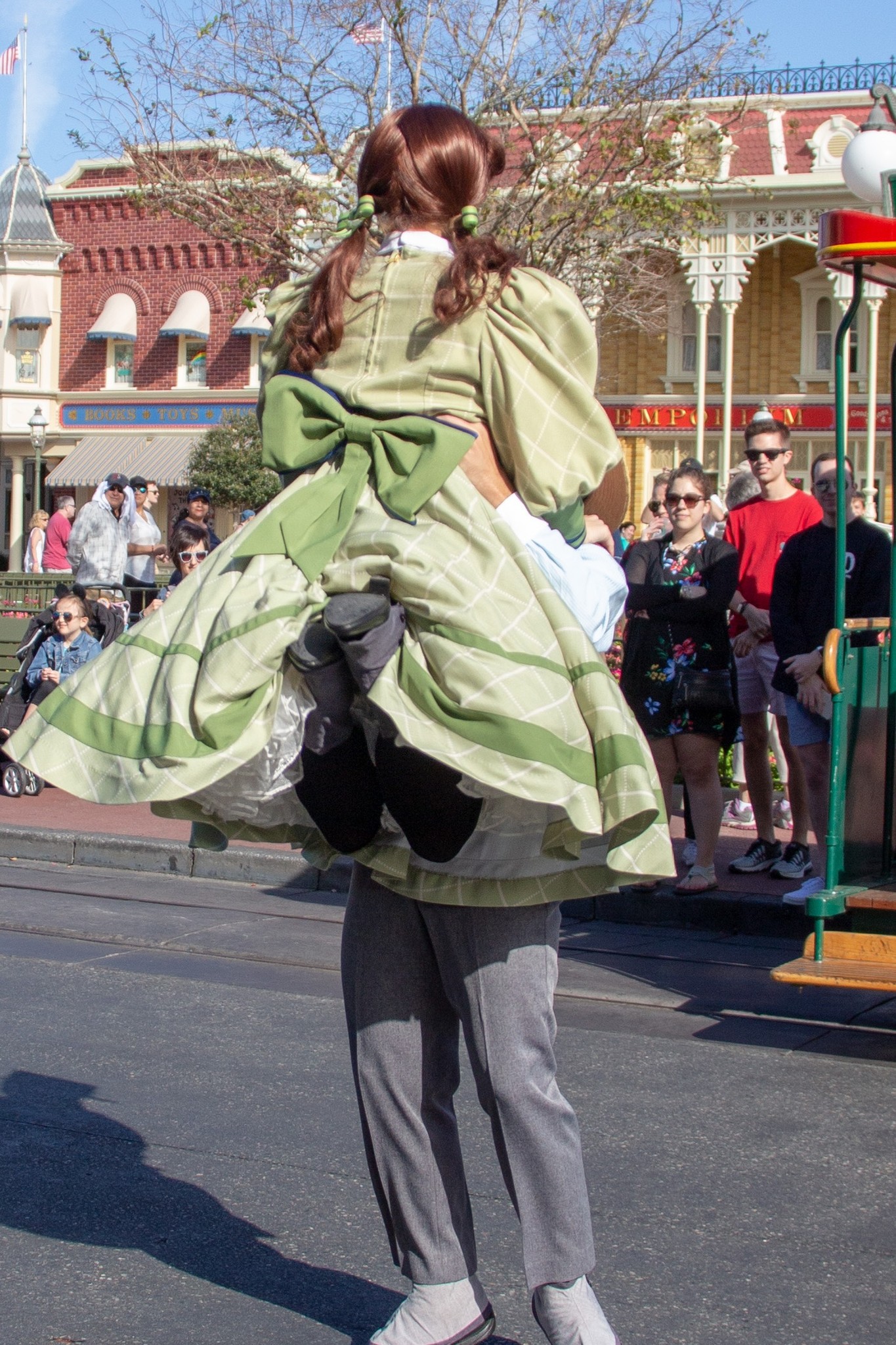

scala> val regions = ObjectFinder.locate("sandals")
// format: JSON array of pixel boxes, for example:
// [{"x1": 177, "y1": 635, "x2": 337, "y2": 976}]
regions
[
  {"x1": 674, "y1": 865, "x2": 719, "y2": 895},
  {"x1": 630, "y1": 881, "x2": 659, "y2": 891}
]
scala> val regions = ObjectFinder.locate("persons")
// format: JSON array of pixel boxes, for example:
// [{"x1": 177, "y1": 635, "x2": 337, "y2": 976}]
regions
[
  {"x1": 1, "y1": 103, "x2": 677, "y2": 907},
  {"x1": 0, "y1": 583, "x2": 102, "y2": 742},
  {"x1": 340, "y1": 413, "x2": 630, "y2": 1345},
  {"x1": 65, "y1": 473, "x2": 168, "y2": 621},
  {"x1": 237, "y1": 509, "x2": 255, "y2": 531},
  {"x1": 172, "y1": 507, "x2": 189, "y2": 527},
  {"x1": 24, "y1": 496, "x2": 76, "y2": 573},
  {"x1": 599, "y1": 418, "x2": 896, "y2": 904},
  {"x1": 137, "y1": 487, "x2": 222, "y2": 621},
  {"x1": 619, "y1": 521, "x2": 637, "y2": 550}
]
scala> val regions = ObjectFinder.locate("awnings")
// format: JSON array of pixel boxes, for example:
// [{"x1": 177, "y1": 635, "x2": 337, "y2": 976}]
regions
[
  {"x1": 45, "y1": 436, "x2": 206, "y2": 486},
  {"x1": 7, "y1": 275, "x2": 273, "y2": 341}
]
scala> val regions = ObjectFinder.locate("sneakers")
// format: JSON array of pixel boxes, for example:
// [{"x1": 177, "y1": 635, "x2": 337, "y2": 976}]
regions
[
  {"x1": 783, "y1": 875, "x2": 825, "y2": 904},
  {"x1": 772, "y1": 800, "x2": 794, "y2": 829},
  {"x1": 722, "y1": 797, "x2": 757, "y2": 831},
  {"x1": 728, "y1": 837, "x2": 782, "y2": 872},
  {"x1": 682, "y1": 837, "x2": 699, "y2": 869},
  {"x1": 771, "y1": 844, "x2": 811, "y2": 879}
]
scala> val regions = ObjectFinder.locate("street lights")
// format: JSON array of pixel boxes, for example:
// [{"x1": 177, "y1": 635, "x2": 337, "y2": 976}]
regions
[{"x1": 27, "y1": 406, "x2": 49, "y2": 517}]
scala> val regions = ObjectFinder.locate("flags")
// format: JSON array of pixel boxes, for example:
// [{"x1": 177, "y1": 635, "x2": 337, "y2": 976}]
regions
[
  {"x1": 0, "y1": 35, "x2": 21, "y2": 75},
  {"x1": 351, "y1": 20, "x2": 384, "y2": 44}
]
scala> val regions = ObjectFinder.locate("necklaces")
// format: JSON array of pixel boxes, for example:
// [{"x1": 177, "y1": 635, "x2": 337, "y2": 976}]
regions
[{"x1": 238, "y1": 523, "x2": 243, "y2": 526}]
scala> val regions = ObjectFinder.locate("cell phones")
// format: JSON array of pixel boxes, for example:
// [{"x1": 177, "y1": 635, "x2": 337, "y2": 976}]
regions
[{"x1": 798, "y1": 688, "x2": 834, "y2": 719}]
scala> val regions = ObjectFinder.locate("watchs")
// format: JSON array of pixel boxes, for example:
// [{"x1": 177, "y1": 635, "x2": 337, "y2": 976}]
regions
[
  {"x1": 683, "y1": 586, "x2": 688, "y2": 599},
  {"x1": 736, "y1": 601, "x2": 749, "y2": 614}
]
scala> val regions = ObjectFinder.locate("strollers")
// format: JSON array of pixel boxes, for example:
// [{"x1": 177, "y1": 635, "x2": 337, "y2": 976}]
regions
[{"x1": 0, "y1": 580, "x2": 132, "y2": 798}]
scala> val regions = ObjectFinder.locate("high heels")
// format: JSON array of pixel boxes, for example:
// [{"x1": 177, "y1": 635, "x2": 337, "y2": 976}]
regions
[
  {"x1": 284, "y1": 618, "x2": 341, "y2": 673},
  {"x1": 321, "y1": 574, "x2": 396, "y2": 638}
]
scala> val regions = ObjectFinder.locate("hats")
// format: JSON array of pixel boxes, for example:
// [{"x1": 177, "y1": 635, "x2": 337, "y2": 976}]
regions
[
  {"x1": 187, "y1": 487, "x2": 210, "y2": 503},
  {"x1": 129, "y1": 476, "x2": 147, "y2": 488},
  {"x1": 105, "y1": 473, "x2": 130, "y2": 488},
  {"x1": 240, "y1": 509, "x2": 255, "y2": 522}
]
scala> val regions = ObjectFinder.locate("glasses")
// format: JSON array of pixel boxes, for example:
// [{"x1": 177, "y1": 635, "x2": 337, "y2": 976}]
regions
[
  {"x1": 147, "y1": 490, "x2": 160, "y2": 495},
  {"x1": 743, "y1": 448, "x2": 791, "y2": 462},
  {"x1": 39, "y1": 518, "x2": 48, "y2": 522},
  {"x1": 175, "y1": 550, "x2": 209, "y2": 563},
  {"x1": 815, "y1": 479, "x2": 850, "y2": 492},
  {"x1": 109, "y1": 485, "x2": 124, "y2": 494},
  {"x1": 647, "y1": 500, "x2": 664, "y2": 512},
  {"x1": 132, "y1": 487, "x2": 147, "y2": 494},
  {"x1": 52, "y1": 612, "x2": 86, "y2": 621},
  {"x1": 67, "y1": 504, "x2": 76, "y2": 508},
  {"x1": 666, "y1": 494, "x2": 707, "y2": 508}
]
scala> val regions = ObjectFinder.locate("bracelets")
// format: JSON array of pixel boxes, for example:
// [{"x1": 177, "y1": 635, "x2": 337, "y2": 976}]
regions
[
  {"x1": 33, "y1": 560, "x2": 38, "y2": 563},
  {"x1": 687, "y1": 586, "x2": 694, "y2": 600},
  {"x1": 151, "y1": 545, "x2": 154, "y2": 553}
]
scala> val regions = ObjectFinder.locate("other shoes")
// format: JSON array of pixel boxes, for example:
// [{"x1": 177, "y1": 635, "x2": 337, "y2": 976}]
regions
[
  {"x1": 531, "y1": 1293, "x2": 538, "y2": 1322},
  {"x1": 448, "y1": 1309, "x2": 497, "y2": 1345}
]
